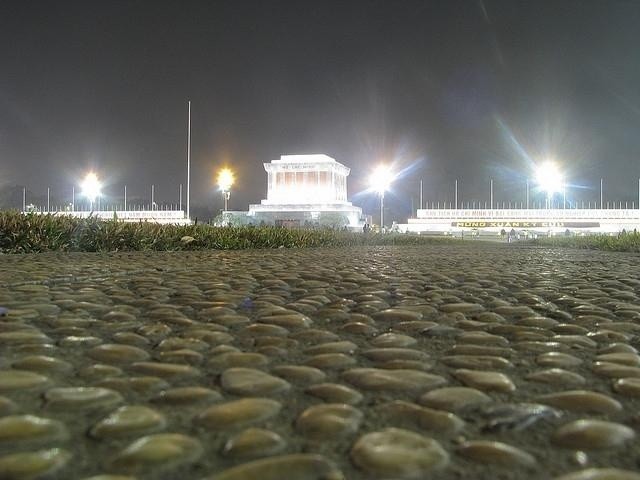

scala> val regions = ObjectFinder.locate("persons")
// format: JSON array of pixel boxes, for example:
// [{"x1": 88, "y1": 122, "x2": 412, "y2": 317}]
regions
[
  {"x1": 622, "y1": 228, "x2": 626, "y2": 233},
  {"x1": 565, "y1": 229, "x2": 570, "y2": 236},
  {"x1": 498, "y1": 227, "x2": 537, "y2": 243},
  {"x1": 362, "y1": 223, "x2": 409, "y2": 234}
]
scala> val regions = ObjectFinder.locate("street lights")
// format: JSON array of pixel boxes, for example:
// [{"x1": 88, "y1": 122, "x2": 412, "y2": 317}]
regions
[
  {"x1": 368, "y1": 163, "x2": 394, "y2": 232},
  {"x1": 218, "y1": 164, "x2": 234, "y2": 211}
]
